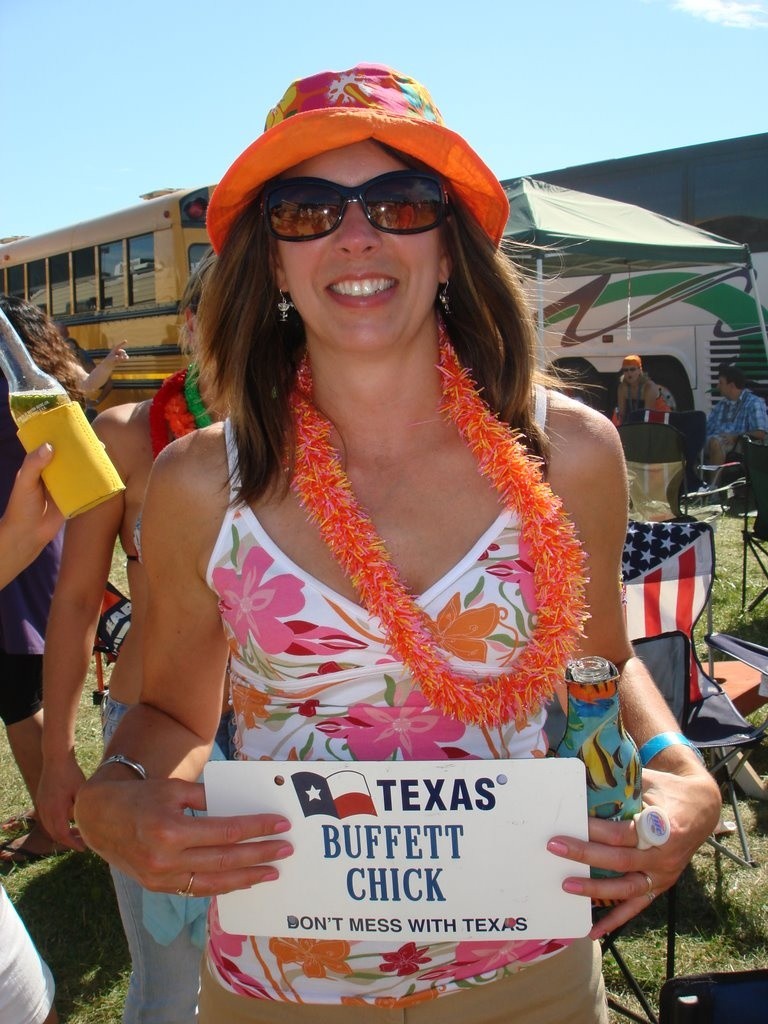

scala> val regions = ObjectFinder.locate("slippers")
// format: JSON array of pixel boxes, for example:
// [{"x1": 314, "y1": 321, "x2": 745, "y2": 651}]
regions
[
  {"x1": 0, "y1": 833, "x2": 71, "y2": 862},
  {"x1": 4, "y1": 812, "x2": 39, "y2": 832}
]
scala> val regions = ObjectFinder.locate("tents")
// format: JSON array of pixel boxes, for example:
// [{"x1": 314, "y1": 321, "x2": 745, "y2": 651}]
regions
[{"x1": 502, "y1": 176, "x2": 768, "y2": 384}]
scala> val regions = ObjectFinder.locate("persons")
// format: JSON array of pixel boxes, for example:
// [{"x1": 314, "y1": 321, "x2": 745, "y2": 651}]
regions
[
  {"x1": 0, "y1": 247, "x2": 235, "y2": 1024},
  {"x1": 693, "y1": 368, "x2": 768, "y2": 505},
  {"x1": 74, "y1": 66, "x2": 722, "y2": 1024},
  {"x1": 617, "y1": 356, "x2": 657, "y2": 425}
]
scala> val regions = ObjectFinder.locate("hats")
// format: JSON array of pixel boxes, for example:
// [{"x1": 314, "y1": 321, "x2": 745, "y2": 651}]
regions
[
  {"x1": 622, "y1": 355, "x2": 641, "y2": 367},
  {"x1": 205, "y1": 66, "x2": 508, "y2": 256}
]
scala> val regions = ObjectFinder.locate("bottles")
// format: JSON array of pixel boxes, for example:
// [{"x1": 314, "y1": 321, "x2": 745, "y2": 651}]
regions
[
  {"x1": 0, "y1": 311, "x2": 129, "y2": 522},
  {"x1": 612, "y1": 405, "x2": 622, "y2": 427},
  {"x1": 551, "y1": 654, "x2": 647, "y2": 916}
]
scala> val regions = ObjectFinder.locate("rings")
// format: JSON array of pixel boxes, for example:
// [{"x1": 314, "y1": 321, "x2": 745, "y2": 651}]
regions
[
  {"x1": 633, "y1": 806, "x2": 670, "y2": 850},
  {"x1": 178, "y1": 876, "x2": 193, "y2": 896},
  {"x1": 644, "y1": 875, "x2": 656, "y2": 901},
  {"x1": 125, "y1": 354, "x2": 127, "y2": 356}
]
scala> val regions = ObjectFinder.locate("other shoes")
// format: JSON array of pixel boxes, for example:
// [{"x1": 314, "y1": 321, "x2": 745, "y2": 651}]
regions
[{"x1": 694, "y1": 488, "x2": 721, "y2": 504}]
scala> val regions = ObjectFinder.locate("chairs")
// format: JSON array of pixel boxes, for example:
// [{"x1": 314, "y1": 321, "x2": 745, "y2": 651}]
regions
[{"x1": 601, "y1": 409, "x2": 768, "y2": 1024}]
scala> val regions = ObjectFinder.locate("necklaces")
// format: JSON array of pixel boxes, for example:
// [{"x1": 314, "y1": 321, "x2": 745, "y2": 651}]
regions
[
  {"x1": 161, "y1": 376, "x2": 196, "y2": 439},
  {"x1": 150, "y1": 368, "x2": 187, "y2": 461},
  {"x1": 287, "y1": 322, "x2": 590, "y2": 728},
  {"x1": 185, "y1": 362, "x2": 216, "y2": 428}
]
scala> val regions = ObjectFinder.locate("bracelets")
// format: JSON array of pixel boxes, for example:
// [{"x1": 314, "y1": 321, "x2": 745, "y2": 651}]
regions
[
  {"x1": 640, "y1": 731, "x2": 707, "y2": 767},
  {"x1": 96, "y1": 755, "x2": 147, "y2": 780}
]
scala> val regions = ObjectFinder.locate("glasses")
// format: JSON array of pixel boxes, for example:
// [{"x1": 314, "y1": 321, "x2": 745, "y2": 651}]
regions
[
  {"x1": 259, "y1": 170, "x2": 448, "y2": 241},
  {"x1": 623, "y1": 368, "x2": 636, "y2": 371}
]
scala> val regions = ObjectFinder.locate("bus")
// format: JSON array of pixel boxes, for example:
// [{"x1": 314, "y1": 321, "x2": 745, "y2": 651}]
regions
[
  {"x1": 494, "y1": 129, "x2": 767, "y2": 427},
  {"x1": 1, "y1": 185, "x2": 226, "y2": 417}
]
